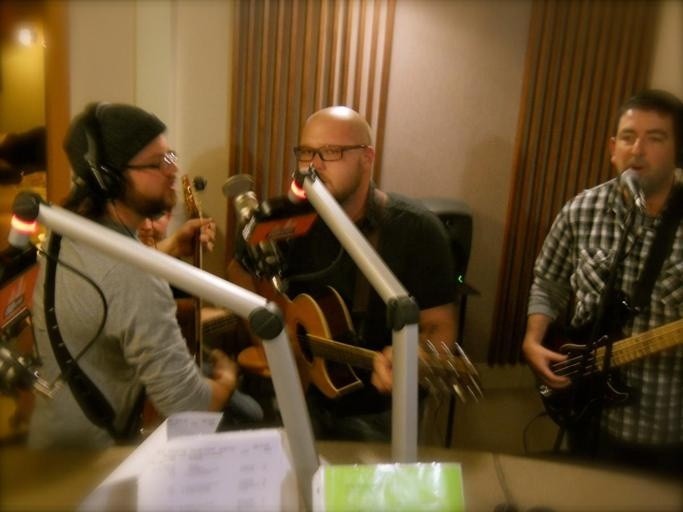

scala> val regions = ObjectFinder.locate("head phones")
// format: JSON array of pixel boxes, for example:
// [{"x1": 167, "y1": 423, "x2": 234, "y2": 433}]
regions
[{"x1": 77, "y1": 102, "x2": 126, "y2": 201}]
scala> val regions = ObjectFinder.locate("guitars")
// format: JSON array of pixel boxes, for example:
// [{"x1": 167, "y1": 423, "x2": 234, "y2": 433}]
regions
[
  {"x1": 220, "y1": 253, "x2": 485, "y2": 410},
  {"x1": 136, "y1": 172, "x2": 206, "y2": 443},
  {"x1": 534, "y1": 292, "x2": 683, "y2": 426}
]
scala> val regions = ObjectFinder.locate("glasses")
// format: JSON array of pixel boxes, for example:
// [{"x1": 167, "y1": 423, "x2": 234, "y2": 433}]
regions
[
  {"x1": 121, "y1": 150, "x2": 179, "y2": 173},
  {"x1": 293, "y1": 144, "x2": 366, "y2": 162}
]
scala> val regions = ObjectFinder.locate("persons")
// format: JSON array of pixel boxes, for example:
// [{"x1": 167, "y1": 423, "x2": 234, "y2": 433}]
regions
[
  {"x1": 136, "y1": 204, "x2": 193, "y2": 299},
  {"x1": 519, "y1": 88, "x2": 682, "y2": 479},
  {"x1": 23, "y1": 101, "x2": 240, "y2": 451},
  {"x1": 223, "y1": 104, "x2": 458, "y2": 444}
]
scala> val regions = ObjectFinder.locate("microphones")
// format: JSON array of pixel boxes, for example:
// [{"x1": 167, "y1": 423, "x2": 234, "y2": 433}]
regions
[
  {"x1": 619, "y1": 168, "x2": 647, "y2": 213},
  {"x1": 288, "y1": 169, "x2": 308, "y2": 206},
  {"x1": 8, "y1": 212, "x2": 37, "y2": 248},
  {"x1": 233, "y1": 190, "x2": 284, "y2": 295}
]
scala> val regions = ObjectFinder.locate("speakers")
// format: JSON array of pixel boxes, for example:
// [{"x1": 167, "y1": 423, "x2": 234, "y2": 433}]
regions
[{"x1": 416, "y1": 196, "x2": 473, "y2": 286}]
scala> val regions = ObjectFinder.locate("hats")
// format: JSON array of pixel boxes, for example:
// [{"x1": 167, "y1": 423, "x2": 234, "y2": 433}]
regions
[{"x1": 63, "y1": 102, "x2": 166, "y2": 194}]
